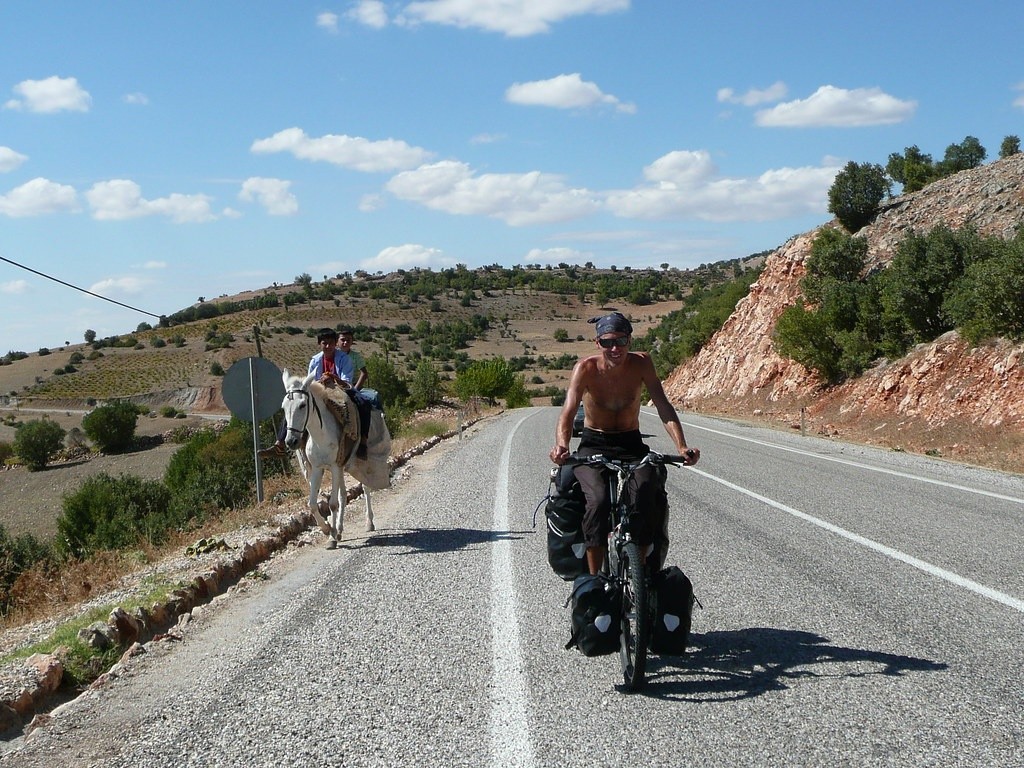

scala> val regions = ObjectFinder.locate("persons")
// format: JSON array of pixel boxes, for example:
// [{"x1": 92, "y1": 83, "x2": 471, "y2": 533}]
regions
[
  {"x1": 549, "y1": 312, "x2": 700, "y2": 576},
  {"x1": 256, "y1": 328, "x2": 372, "y2": 461},
  {"x1": 335, "y1": 330, "x2": 368, "y2": 391}
]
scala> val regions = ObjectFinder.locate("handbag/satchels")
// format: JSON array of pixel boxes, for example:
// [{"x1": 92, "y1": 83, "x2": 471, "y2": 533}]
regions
[
  {"x1": 532, "y1": 493, "x2": 588, "y2": 580},
  {"x1": 648, "y1": 565, "x2": 704, "y2": 654},
  {"x1": 561, "y1": 569, "x2": 624, "y2": 658}
]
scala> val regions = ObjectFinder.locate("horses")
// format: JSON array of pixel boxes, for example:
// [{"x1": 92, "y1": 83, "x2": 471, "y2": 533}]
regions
[{"x1": 282, "y1": 366, "x2": 391, "y2": 551}]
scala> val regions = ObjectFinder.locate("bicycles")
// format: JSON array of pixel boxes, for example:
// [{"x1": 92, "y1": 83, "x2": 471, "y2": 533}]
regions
[{"x1": 556, "y1": 451, "x2": 697, "y2": 692}]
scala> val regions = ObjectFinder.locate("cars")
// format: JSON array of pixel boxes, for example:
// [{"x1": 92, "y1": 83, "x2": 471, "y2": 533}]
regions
[{"x1": 572, "y1": 401, "x2": 587, "y2": 439}]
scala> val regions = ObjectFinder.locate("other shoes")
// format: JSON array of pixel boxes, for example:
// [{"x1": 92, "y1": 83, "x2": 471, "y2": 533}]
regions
[{"x1": 356, "y1": 442, "x2": 368, "y2": 460}]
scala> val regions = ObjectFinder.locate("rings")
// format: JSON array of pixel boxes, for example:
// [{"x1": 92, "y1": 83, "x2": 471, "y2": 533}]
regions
[{"x1": 697, "y1": 457, "x2": 700, "y2": 460}]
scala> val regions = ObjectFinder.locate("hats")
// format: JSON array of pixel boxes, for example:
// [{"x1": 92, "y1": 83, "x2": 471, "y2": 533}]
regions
[{"x1": 596, "y1": 312, "x2": 633, "y2": 335}]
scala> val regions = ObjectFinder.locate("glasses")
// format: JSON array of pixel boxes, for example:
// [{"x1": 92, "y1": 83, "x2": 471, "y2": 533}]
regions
[{"x1": 598, "y1": 335, "x2": 630, "y2": 348}]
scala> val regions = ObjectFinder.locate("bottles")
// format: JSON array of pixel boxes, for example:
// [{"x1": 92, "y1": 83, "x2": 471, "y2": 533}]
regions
[{"x1": 607, "y1": 532, "x2": 613, "y2": 574}]
